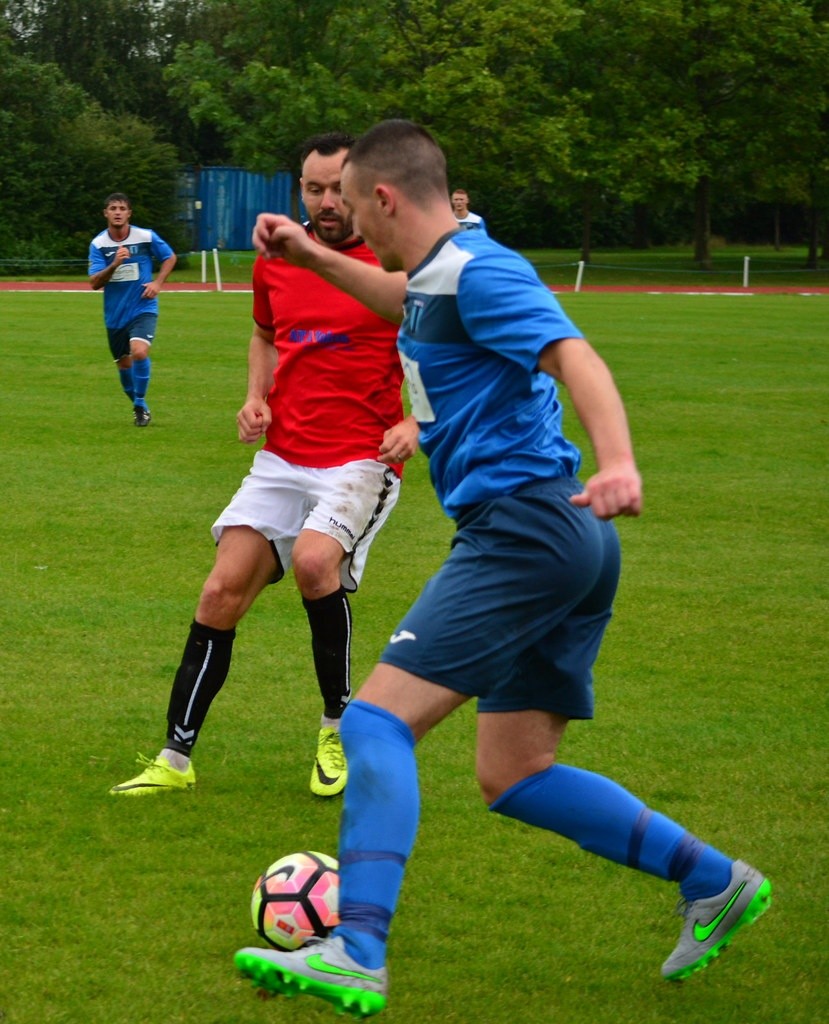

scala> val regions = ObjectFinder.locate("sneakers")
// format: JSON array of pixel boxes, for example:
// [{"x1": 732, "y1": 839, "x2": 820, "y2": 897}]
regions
[
  {"x1": 108, "y1": 759, "x2": 196, "y2": 795},
  {"x1": 657, "y1": 857, "x2": 774, "y2": 981},
  {"x1": 308, "y1": 725, "x2": 348, "y2": 796},
  {"x1": 231, "y1": 933, "x2": 390, "y2": 1020},
  {"x1": 132, "y1": 405, "x2": 152, "y2": 427}
]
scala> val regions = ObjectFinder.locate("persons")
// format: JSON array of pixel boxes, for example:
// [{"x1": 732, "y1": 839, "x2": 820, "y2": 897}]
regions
[
  {"x1": 87, "y1": 191, "x2": 177, "y2": 425},
  {"x1": 450, "y1": 187, "x2": 491, "y2": 233},
  {"x1": 107, "y1": 130, "x2": 419, "y2": 805},
  {"x1": 237, "y1": 119, "x2": 775, "y2": 1016}
]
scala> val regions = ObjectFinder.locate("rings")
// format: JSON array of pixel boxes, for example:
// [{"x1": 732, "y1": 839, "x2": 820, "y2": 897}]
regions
[{"x1": 397, "y1": 461, "x2": 423, "y2": 464}]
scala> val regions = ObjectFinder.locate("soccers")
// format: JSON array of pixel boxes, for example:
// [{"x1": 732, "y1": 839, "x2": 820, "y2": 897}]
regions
[{"x1": 249, "y1": 849, "x2": 343, "y2": 952}]
